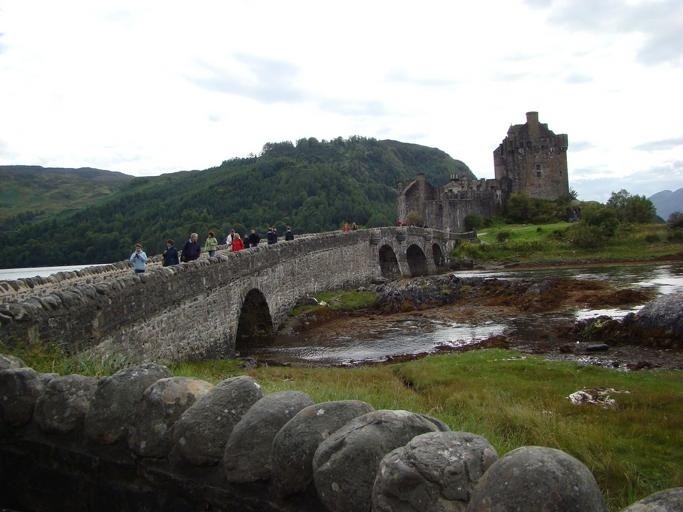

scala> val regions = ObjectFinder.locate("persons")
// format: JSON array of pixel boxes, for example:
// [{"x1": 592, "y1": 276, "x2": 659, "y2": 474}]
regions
[
  {"x1": 129, "y1": 242, "x2": 149, "y2": 273},
  {"x1": 343, "y1": 222, "x2": 350, "y2": 233},
  {"x1": 204, "y1": 231, "x2": 218, "y2": 256},
  {"x1": 225, "y1": 228, "x2": 236, "y2": 246},
  {"x1": 273, "y1": 228, "x2": 279, "y2": 244},
  {"x1": 267, "y1": 227, "x2": 274, "y2": 244},
  {"x1": 161, "y1": 239, "x2": 180, "y2": 266},
  {"x1": 352, "y1": 222, "x2": 358, "y2": 231},
  {"x1": 181, "y1": 232, "x2": 201, "y2": 262},
  {"x1": 248, "y1": 229, "x2": 261, "y2": 248},
  {"x1": 228, "y1": 232, "x2": 244, "y2": 251},
  {"x1": 243, "y1": 234, "x2": 251, "y2": 250},
  {"x1": 285, "y1": 227, "x2": 294, "y2": 241}
]
